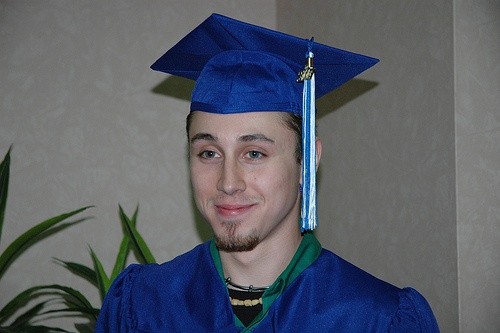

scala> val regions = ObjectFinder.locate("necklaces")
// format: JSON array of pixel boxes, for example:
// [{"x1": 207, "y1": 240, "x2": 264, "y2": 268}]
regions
[{"x1": 224, "y1": 277, "x2": 269, "y2": 291}]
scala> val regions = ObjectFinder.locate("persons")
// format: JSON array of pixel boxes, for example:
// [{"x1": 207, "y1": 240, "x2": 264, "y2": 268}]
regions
[{"x1": 93, "y1": 14, "x2": 441, "y2": 333}]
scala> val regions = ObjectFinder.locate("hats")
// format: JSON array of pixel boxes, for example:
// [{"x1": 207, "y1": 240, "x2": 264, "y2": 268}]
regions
[{"x1": 150, "y1": 12, "x2": 380, "y2": 233}]
